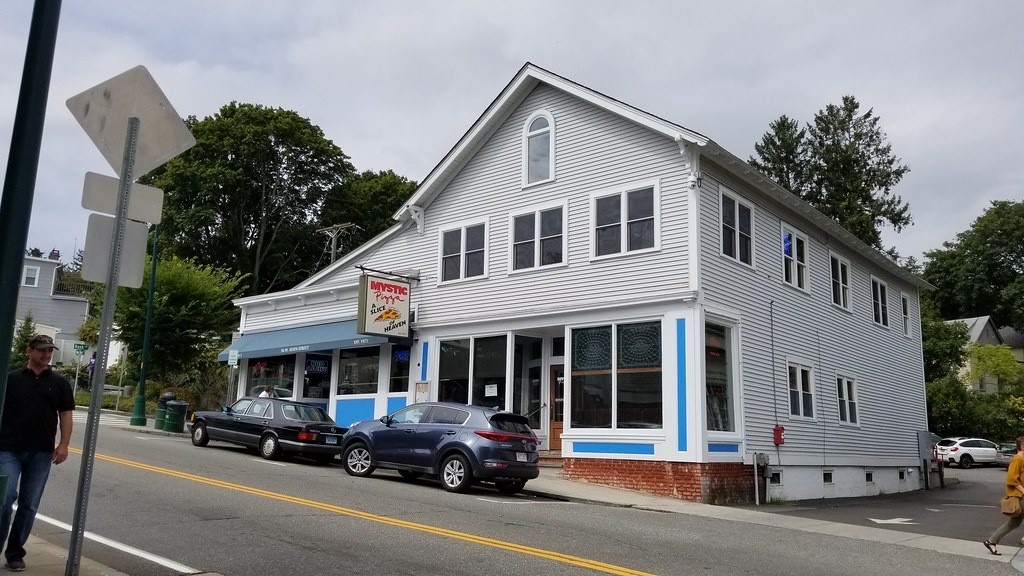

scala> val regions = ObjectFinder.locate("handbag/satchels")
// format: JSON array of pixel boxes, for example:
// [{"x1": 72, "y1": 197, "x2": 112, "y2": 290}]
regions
[{"x1": 1000, "y1": 495, "x2": 1022, "y2": 517}]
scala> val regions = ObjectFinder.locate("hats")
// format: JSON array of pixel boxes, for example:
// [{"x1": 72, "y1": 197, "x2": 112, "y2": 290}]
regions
[{"x1": 28, "y1": 334, "x2": 59, "y2": 350}]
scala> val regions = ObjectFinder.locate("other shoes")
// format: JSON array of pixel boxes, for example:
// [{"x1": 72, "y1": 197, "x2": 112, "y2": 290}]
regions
[{"x1": 4, "y1": 558, "x2": 26, "y2": 571}]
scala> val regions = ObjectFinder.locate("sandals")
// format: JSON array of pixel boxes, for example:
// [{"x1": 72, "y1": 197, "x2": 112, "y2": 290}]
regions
[{"x1": 983, "y1": 538, "x2": 1002, "y2": 555}]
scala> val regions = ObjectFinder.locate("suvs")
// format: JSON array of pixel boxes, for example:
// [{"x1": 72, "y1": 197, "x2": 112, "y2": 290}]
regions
[
  {"x1": 933, "y1": 436, "x2": 999, "y2": 469},
  {"x1": 339, "y1": 401, "x2": 540, "y2": 495}
]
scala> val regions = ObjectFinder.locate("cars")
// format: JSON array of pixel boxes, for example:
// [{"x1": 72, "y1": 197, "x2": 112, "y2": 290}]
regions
[
  {"x1": 247, "y1": 377, "x2": 293, "y2": 400},
  {"x1": 190, "y1": 395, "x2": 348, "y2": 467},
  {"x1": 995, "y1": 442, "x2": 1019, "y2": 469}
]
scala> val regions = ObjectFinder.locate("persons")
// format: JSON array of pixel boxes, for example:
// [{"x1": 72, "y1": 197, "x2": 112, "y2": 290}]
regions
[
  {"x1": 0, "y1": 335, "x2": 75, "y2": 573},
  {"x1": 259, "y1": 386, "x2": 274, "y2": 398},
  {"x1": 85, "y1": 352, "x2": 96, "y2": 391},
  {"x1": 983, "y1": 438, "x2": 1024, "y2": 555}
]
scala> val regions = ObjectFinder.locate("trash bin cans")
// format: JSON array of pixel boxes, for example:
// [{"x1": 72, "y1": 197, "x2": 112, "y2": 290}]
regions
[
  {"x1": 163, "y1": 402, "x2": 189, "y2": 433},
  {"x1": 154, "y1": 395, "x2": 178, "y2": 429}
]
